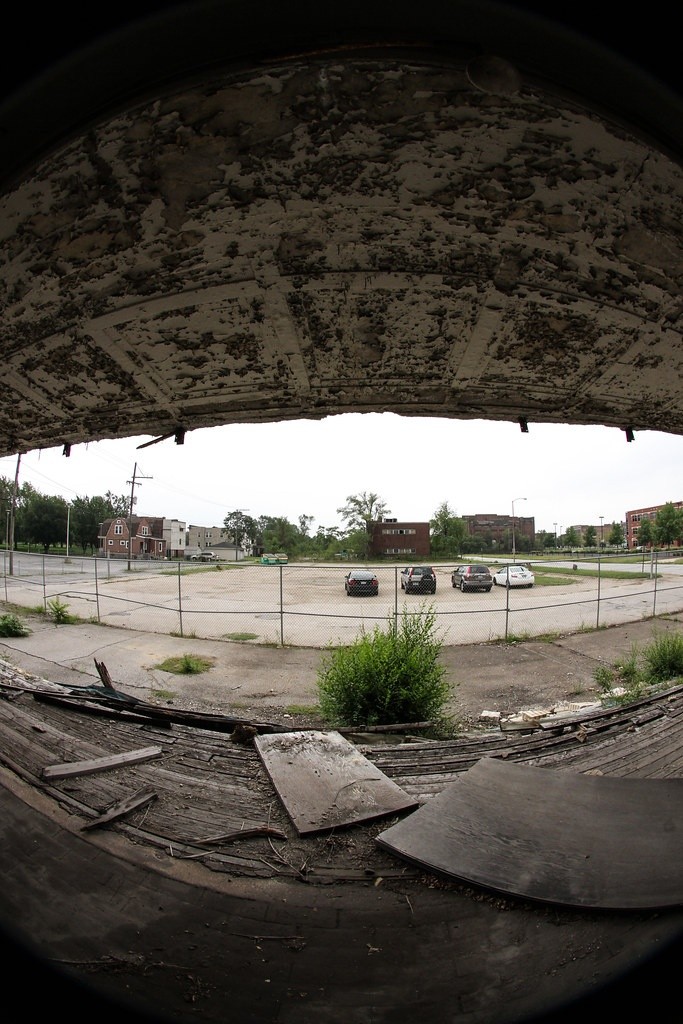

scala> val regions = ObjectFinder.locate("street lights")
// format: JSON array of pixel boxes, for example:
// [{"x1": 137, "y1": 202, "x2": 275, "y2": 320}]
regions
[
  {"x1": 5, "y1": 510, "x2": 10, "y2": 549},
  {"x1": 511, "y1": 497, "x2": 527, "y2": 562},
  {"x1": 99, "y1": 523, "x2": 103, "y2": 546},
  {"x1": 599, "y1": 516, "x2": 604, "y2": 541},
  {"x1": 560, "y1": 526, "x2": 562, "y2": 545}
]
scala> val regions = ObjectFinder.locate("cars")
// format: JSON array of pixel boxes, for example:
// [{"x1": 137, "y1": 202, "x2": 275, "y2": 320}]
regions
[
  {"x1": 345, "y1": 572, "x2": 379, "y2": 597},
  {"x1": 636, "y1": 546, "x2": 647, "y2": 550},
  {"x1": 493, "y1": 566, "x2": 535, "y2": 590}
]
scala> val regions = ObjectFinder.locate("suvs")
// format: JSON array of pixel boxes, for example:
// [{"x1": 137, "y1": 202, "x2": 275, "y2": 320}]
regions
[
  {"x1": 451, "y1": 565, "x2": 493, "y2": 593},
  {"x1": 400, "y1": 566, "x2": 436, "y2": 594},
  {"x1": 190, "y1": 552, "x2": 216, "y2": 561}
]
